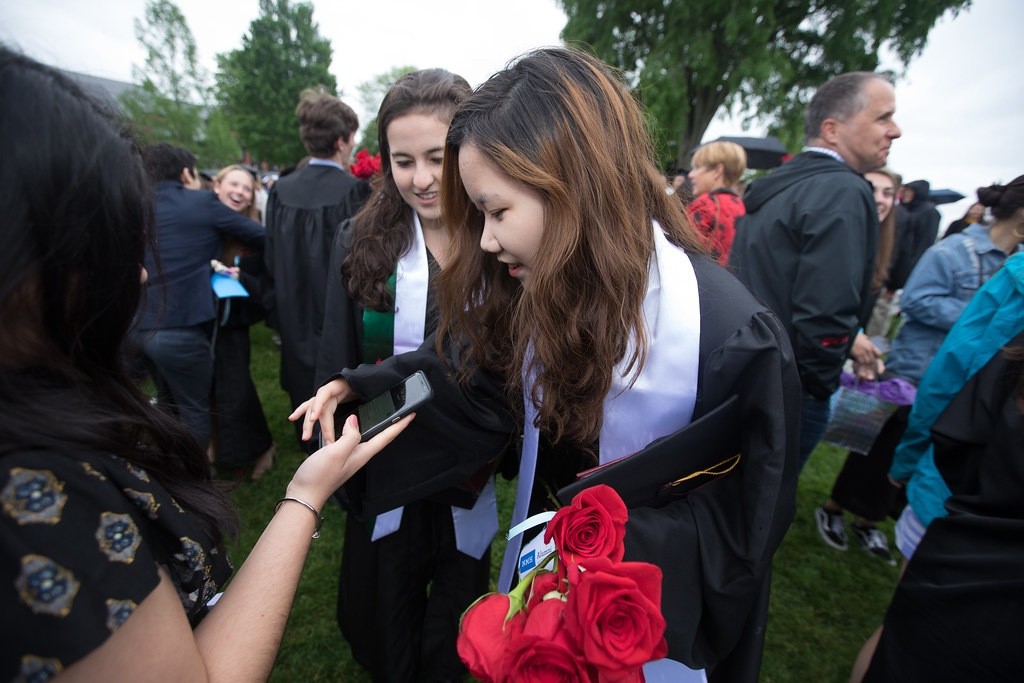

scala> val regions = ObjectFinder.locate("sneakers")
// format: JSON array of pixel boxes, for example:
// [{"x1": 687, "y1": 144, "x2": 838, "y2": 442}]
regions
[
  {"x1": 815, "y1": 504, "x2": 850, "y2": 551},
  {"x1": 853, "y1": 520, "x2": 897, "y2": 565}
]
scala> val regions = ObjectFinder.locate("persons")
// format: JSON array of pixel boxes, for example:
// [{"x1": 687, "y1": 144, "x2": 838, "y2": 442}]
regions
[
  {"x1": 0, "y1": 43, "x2": 417, "y2": 683},
  {"x1": 734, "y1": 65, "x2": 903, "y2": 502},
  {"x1": 283, "y1": 49, "x2": 789, "y2": 679},
  {"x1": 327, "y1": 68, "x2": 525, "y2": 680},
  {"x1": 111, "y1": 84, "x2": 1024, "y2": 680}
]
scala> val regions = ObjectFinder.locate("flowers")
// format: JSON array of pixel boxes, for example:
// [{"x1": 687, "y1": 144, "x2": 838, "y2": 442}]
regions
[
  {"x1": 348, "y1": 149, "x2": 384, "y2": 180},
  {"x1": 458, "y1": 484, "x2": 668, "y2": 683}
]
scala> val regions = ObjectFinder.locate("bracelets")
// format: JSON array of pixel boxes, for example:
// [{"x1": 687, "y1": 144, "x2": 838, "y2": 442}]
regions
[{"x1": 272, "y1": 497, "x2": 326, "y2": 541}]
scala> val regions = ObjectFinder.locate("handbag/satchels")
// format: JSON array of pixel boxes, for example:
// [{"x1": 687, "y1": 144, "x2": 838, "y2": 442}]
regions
[
  {"x1": 556, "y1": 397, "x2": 771, "y2": 517},
  {"x1": 821, "y1": 362, "x2": 892, "y2": 456}
]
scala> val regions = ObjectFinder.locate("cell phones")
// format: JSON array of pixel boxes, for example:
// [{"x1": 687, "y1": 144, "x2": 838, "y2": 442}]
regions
[{"x1": 319, "y1": 370, "x2": 434, "y2": 450}]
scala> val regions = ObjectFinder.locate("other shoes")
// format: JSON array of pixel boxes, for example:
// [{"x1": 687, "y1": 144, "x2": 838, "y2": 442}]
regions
[{"x1": 248, "y1": 442, "x2": 277, "y2": 479}]
